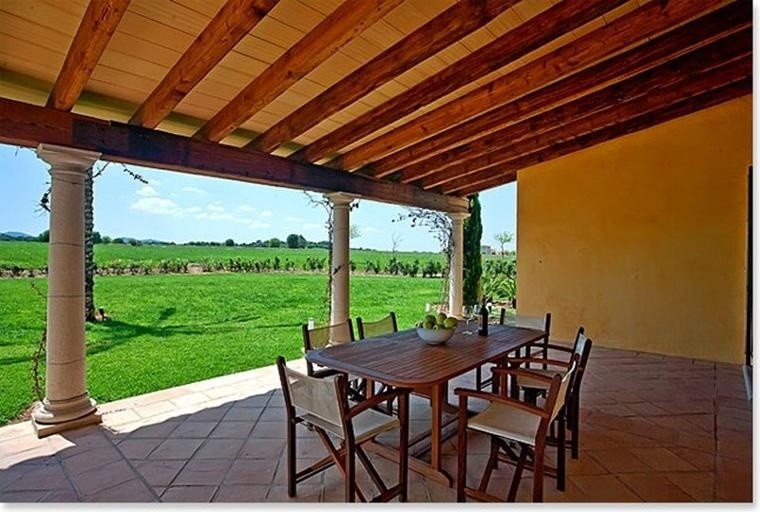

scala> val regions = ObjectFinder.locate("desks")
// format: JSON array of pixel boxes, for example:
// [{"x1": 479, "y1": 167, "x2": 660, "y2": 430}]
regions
[{"x1": 304, "y1": 320, "x2": 549, "y2": 488}]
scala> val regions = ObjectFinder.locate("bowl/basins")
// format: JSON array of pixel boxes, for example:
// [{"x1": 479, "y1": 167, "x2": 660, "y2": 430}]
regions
[{"x1": 415, "y1": 327, "x2": 455, "y2": 343}]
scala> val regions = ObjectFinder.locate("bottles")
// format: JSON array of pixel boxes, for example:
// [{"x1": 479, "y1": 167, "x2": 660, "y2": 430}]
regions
[{"x1": 479, "y1": 297, "x2": 489, "y2": 335}]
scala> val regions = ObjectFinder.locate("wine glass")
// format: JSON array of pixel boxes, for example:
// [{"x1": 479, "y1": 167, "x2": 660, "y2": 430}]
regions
[{"x1": 461, "y1": 305, "x2": 484, "y2": 335}]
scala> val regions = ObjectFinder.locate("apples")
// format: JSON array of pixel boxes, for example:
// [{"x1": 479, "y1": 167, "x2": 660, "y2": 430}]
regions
[{"x1": 415, "y1": 313, "x2": 457, "y2": 330}]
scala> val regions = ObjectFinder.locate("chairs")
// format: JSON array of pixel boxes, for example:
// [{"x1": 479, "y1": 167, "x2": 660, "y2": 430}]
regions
[
  {"x1": 302, "y1": 318, "x2": 375, "y2": 430},
  {"x1": 453, "y1": 353, "x2": 580, "y2": 503},
  {"x1": 507, "y1": 327, "x2": 592, "y2": 459},
  {"x1": 499, "y1": 308, "x2": 551, "y2": 398},
  {"x1": 276, "y1": 356, "x2": 414, "y2": 502},
  {"x1": 356, "y1": 311, "x2": 398, "y2": 415}
]
